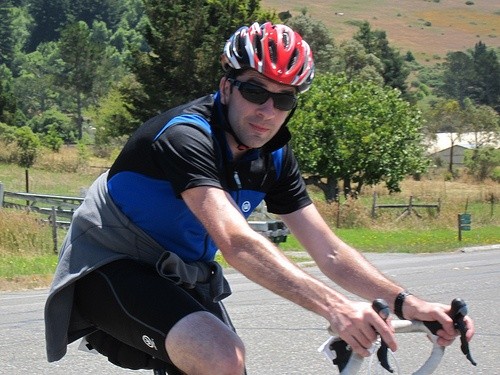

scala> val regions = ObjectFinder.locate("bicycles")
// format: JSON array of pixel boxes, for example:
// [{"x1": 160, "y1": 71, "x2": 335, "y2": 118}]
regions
[{"x1": 75, "y1": 295, "x2": 479, "y2": 374}]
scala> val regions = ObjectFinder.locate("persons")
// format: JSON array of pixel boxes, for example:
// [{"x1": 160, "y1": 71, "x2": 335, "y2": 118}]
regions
[{"x1": 44, "y1": 22, "x2": 476, "y2": 375}]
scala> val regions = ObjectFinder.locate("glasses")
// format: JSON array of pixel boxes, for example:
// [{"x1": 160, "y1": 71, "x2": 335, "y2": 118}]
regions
[{"x1": 227, "y1": 77, "x2": 297, "y2": 110}]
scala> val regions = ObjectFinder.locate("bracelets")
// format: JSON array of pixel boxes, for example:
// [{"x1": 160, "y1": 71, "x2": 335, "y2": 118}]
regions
[{"x1": 394, "y1": 290, "x2": 413, "y2": 321}]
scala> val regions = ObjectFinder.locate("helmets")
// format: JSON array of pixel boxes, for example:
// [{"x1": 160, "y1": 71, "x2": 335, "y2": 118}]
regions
[{"x1": 220, "y1": 21, "x2": 315, "y2": 94}]
